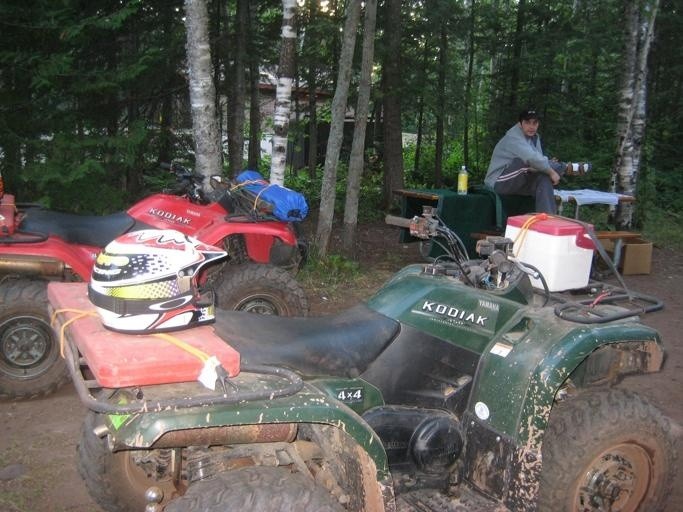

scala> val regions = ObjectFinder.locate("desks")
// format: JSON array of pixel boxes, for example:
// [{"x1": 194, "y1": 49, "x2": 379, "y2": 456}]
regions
[
  {"x1": 392, "y1": 188, "x2": 497, "y2": 258},
  {"x1": 554, "y1": 189, "x2": 637, "y2": 219}
]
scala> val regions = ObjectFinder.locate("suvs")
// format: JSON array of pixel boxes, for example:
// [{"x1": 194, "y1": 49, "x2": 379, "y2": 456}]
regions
[
  {"x1": 43, "y1": 214, "x2": 678, "y2": 512},
  {"x1": 0, "y1": 171, "x2": 310, "y2": 402}
]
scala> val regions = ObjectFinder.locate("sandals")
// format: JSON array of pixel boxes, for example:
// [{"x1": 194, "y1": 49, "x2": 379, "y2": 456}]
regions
[{"x1": 565, "y1": 162, "x2": 592, "y2": 177}]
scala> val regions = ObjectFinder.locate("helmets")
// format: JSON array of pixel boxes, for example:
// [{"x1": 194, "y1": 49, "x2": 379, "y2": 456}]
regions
[{"x1": 87, "y1": 229, "x2": 229, "y2": 334}]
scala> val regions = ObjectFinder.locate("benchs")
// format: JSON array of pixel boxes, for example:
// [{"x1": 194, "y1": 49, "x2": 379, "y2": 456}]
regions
[{"x1": 593, "y1": 231, "x2": 642, "y2": 273}]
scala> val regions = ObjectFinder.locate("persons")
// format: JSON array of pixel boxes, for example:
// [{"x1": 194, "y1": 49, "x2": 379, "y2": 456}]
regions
[{"x1": 484, "y1": 109, "x2": 592, "y2": 215}]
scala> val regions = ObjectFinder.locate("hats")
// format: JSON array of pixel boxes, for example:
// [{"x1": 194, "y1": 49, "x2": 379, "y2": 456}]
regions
[{"x1": 519, "y1": 109, "x2": 543, "y2": 119}]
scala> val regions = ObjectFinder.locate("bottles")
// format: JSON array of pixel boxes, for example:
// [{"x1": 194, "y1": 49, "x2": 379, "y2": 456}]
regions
[{"x1": 458, "y1": 165, "x2": 468, "y2": 196}]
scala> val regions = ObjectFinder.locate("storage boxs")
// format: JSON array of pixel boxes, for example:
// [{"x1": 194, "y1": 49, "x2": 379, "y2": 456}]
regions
[
  {"x1": 605, "y1": 238, "x2": 653, "y2": 275},
  {"x1": 505, "y1": 213, "x2": 594, "y2": 292}
]
